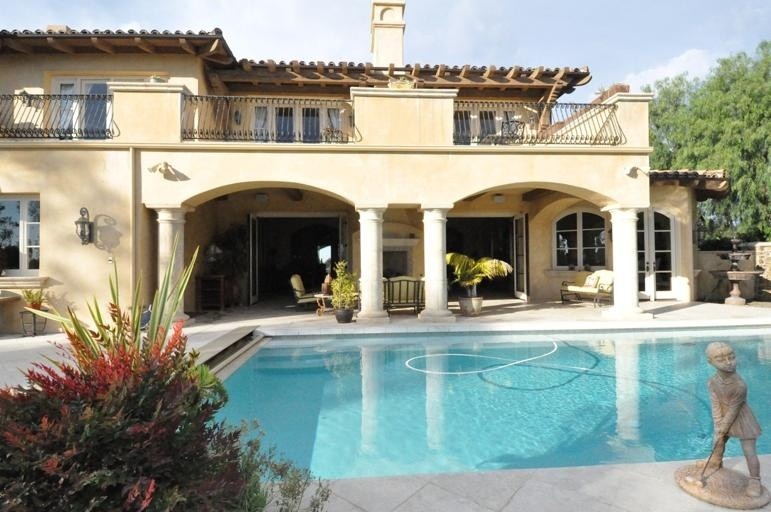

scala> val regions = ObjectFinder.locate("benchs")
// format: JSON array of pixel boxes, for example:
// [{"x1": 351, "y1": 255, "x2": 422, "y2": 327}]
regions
[
  {"x1": 560, "y1": 269, "x2": 613, "y2": 309},
  {"x1": 290, "y1": 274, "x2": 320, "y2": 312},
  {"x1": 383, "y1": 275, "x2": 424, "y2": 317}
]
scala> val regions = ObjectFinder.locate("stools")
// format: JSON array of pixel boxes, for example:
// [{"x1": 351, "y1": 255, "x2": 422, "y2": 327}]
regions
[{"x1": 19, "y1": 309, "x2": 48, "y2": 336}]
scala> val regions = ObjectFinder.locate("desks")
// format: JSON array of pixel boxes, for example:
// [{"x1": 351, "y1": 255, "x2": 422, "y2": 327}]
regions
[{"x1": 196, "y1": 274, "x2": 235, "y2": 312}]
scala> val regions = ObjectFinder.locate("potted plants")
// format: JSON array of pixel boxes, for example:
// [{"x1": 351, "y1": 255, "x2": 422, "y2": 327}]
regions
[
  {"x1": 445, "y1": 252, "x2": 514, "y2": 317},
  {"x1": 22, "y1": 289, "x2": 44, "y2": 310},
  {"x1": 331, "y1": 261, "x2": 361, "y2": 323}
]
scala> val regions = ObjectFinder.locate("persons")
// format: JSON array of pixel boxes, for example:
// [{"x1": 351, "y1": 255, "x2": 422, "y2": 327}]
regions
[{"x1": 697, "y1": 339, "x2": 764, "y2": 500}]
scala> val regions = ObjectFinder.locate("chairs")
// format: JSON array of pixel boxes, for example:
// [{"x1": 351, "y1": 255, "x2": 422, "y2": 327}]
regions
[{"x1": 494, "y1": 119, "x2": 525, "y2": 146}]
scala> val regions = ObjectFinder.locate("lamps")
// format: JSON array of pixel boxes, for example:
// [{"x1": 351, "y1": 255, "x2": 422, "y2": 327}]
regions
[{"x1": 73, "y1": 208, "x2": 93, "y2": 245}]
